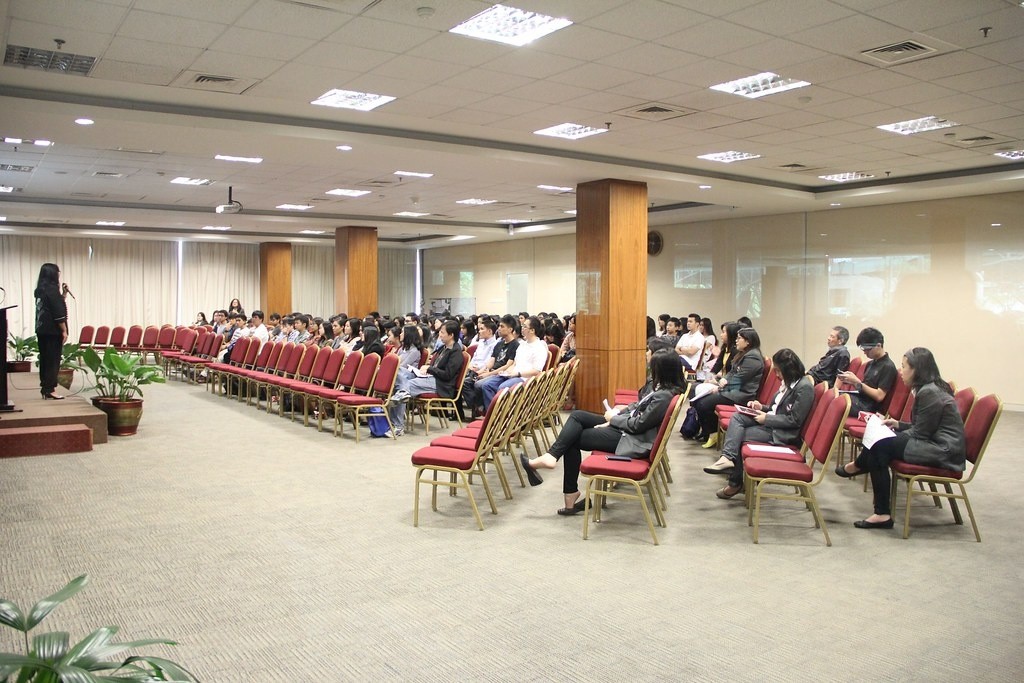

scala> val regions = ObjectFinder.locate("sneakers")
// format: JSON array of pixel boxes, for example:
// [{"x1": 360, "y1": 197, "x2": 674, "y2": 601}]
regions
[{"x1": 384, "y1": 426, "x2": 404, "y2": 437}]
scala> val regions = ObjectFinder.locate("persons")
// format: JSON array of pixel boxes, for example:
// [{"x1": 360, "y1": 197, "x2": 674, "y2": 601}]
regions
[
  {"x1": 179, "y1": 299, "x2": 765, "y2": 437},
  {"x1": 834, "y1": 347, "x2": 967, "y2": 528},
  {"x1": 520, "y1": 348, "x2": 688, "y2": 516},
  {"x1": 694, "y1": 327, "x2": 765, "y2": 449},
  {"x1": 838, "y1": 328, "x2": 896, "y2": 418},
  {"x1": 805, "y1": 326, "x2": 850, "y2": 389},
  {"x1": 703, "y1": 349, "x2": 815, "y2": 499},
  {"x1": 35, "y1": 263, "x2": 68, "y2": 400}
]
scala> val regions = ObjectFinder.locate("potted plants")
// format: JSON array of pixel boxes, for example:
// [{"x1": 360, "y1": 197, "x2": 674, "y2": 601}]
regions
[
  {"x1": 84, "y1": 344, "x2": 165, "y2": 436},
  {"x1": 8, "y1": 334, "x2": 38, "y2": 372},
  {"x1": 58, "y1": 342, "x2": 87, "y2": 390}
]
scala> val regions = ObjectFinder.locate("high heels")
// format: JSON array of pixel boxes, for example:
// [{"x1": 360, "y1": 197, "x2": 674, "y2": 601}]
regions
[
  {"x1": 704, "y1": 461, "x2": 734, "y2": 474},
  {"x1": 716, "y1": 482, "x2": 746, "y2": 500},
  {"x1": 42, "y1": 392, "x2": 65, "y2": 400},
  {"x1": 563, "y1": 399, "x2": 575, "y2": 410}
]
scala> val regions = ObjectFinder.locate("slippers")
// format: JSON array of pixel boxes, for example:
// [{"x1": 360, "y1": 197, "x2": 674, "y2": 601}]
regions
[
  {"x1": 558, "y1": 497, "x2": 593, "y2": 515},
  {"x1": 520, "y1": 452, "x2": 543, "y2": 487}
]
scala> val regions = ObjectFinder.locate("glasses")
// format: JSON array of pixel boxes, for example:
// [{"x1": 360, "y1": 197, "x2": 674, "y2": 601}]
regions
[{"x1": 860, "y1": 345, "x2": 873, "y2": 351}]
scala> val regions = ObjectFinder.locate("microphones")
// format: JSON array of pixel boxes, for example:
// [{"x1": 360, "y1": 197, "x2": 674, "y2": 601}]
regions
[{"x1": 62, "y1": 282, "x2": 76, "y2": 300}]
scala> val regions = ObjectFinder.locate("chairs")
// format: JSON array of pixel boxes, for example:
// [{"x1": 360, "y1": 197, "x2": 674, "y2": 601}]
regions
[
  {"x1": 580, "y1": 394, "x2": 686, "y2": 545},
  {"x1": 613, "y1": 389, "x2": 637, "y2": 406},
  {"x1": 741, "y1": 381, "x2": 851, "y2": 545},
  {"x1": 841, "y1": 357, "x2": 869, "y2": 391},
  {"x1": 714, "y1": 358, "x2": 782, "y2": 450},
  {"x1": 412, "y1": 387, "x2": 512, "y2": 530},
  {"x1": 891, "y1": 394, "x2": 1003, "y2": 541},
  {"x1": 954, "y1": 385, "x2": 977, "y2": 428},
  {"x1": 430, "y1": 383, "x2": 525, "y2": 513},
  {"x1": 849, "y1": 375, "x2": 910, "y2": 491},
  {"x1": 453, "y1": 358, "x2": 581, "y2": 439},
  {"x1": 836, "y1": 368, "x2": 901, "y2": 468},
  {"x1": 79, "y1": 324, "x2": 400, "y2": 444}
]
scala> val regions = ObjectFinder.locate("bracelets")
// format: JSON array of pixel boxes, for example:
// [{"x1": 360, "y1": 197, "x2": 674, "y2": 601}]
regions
[{"x1": 518, "y1": 372, "x2": 521, "y2": 378}]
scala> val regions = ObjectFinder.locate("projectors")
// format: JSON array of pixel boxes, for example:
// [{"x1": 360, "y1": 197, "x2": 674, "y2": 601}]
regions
[{"x1": 216, "y1": 204, "x2": 241, "y2": 214}]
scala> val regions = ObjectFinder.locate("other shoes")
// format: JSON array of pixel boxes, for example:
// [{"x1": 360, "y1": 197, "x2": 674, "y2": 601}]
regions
[
  {"x1": 449, "y1": 412, "x2": 465, "y2": 421},
  {"x1": 854, "y1": 518, "x2": 894, "y2": 529},
  {"x1": 474, "y1": 409, "x2": 485, "y2": 419},
  {"x1": 701, "y1": 432, "x2": 724, "y2": 449},
  {"x1": 835, "y1": 464, "x2": 870, "y2": 477}
]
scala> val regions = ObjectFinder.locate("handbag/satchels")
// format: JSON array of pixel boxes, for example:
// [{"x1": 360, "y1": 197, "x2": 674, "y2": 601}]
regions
[{"x1": 680, "y1": 405, "x2": 701, "y2": 439}]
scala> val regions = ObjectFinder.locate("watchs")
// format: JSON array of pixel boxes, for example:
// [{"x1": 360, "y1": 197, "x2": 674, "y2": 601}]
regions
[{"x1": 855, "y1": 381, "x2": 864, "y2": 391}]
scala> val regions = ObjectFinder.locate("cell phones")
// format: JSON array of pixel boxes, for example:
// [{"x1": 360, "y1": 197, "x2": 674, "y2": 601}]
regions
[
  {"x1": 605, "y1": 455, "x2": 631, "y2": 461},
  {"x1": 837, "y1": 368, "x2": 845, "y2": 374}
]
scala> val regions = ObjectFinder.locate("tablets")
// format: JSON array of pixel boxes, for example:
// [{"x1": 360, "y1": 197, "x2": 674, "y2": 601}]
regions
[{"x1": 733, "y1": 404, "x2": 767, "y2": 417}]
